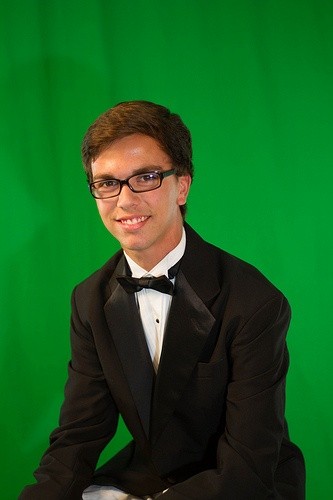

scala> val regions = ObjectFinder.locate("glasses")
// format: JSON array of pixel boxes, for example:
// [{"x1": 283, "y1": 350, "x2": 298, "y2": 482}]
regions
[{"x1": 89, "y1": 168, "x2": 176, "y2": 200}]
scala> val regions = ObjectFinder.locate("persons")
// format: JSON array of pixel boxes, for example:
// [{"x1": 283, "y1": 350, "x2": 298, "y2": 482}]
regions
[{"x1": 14, "y1": 100, "x2": 306, "y2": 499}]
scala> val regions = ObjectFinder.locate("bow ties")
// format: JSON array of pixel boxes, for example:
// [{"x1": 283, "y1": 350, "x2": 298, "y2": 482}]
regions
[{"x1": 116, "y1": 275, "x2": 174, "y2": 296}]
